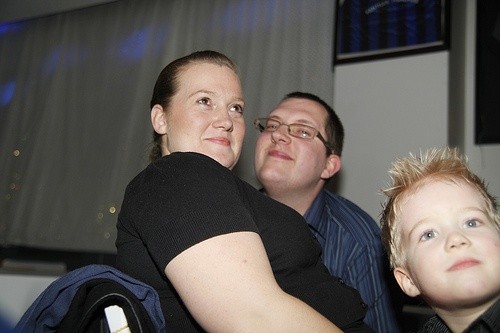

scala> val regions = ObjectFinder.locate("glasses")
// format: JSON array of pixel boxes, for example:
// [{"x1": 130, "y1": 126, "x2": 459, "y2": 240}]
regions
[{"x1": 254, "y1": 118, "x2": 334, "y2": 154}]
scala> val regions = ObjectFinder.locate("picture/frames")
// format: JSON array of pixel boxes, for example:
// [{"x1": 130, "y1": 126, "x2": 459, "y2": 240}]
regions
[{"x1": 334, "y1": 0, "x2": 452, "y2": 66}]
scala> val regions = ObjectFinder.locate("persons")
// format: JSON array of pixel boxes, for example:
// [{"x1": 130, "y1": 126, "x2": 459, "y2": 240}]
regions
[
  {"x1": 377, "y1": 146, "x2": 500, "y2": 333},
  {"x1": 114, "y1": 50, "x2": 375, "y2": 333},
  {"x1": 254, "y1": 92, "x2": 400, "y2": 333}
]
